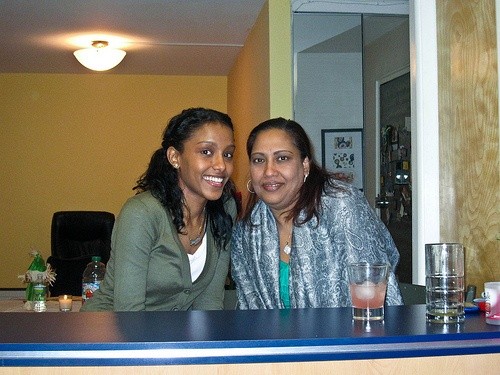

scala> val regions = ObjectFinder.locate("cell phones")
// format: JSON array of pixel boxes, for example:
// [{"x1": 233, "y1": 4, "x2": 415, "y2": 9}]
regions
[{"x1": 464, "y1": 302, "x2": 479, "y2": 313}]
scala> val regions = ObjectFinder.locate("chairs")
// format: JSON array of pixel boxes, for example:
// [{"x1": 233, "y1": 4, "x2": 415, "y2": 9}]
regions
[{"x1": 45, "y1": 210, "x2": 115, "y2": 298}]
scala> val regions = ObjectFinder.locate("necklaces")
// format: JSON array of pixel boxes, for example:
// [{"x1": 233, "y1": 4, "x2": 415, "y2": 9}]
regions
[
  {"x1": 184, "y1": 206, "x2": 205, "y2": 246},
  {"x1": 282, "y1": 228, "x2": 292, "y2": 255}
]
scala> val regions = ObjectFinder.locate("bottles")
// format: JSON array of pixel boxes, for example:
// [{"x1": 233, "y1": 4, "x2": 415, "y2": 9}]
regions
[
  {"x1": 33, "y1": 276, "x2": 47, "y2": 311},
  {"x1": 82, "y1": 257, "x2": 105, "y2": 304}
]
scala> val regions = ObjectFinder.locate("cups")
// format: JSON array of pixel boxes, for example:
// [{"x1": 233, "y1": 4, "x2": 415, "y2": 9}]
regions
[
  {"x1": 346, "y1": 263, "x2": 388, "y2": 321},
  {"x1": 484, "y1": 282, "x2": 500, "y2": 325},
  {"x1": 59, "y1": 295, "x2": 72, "y2": 312},
  {"x1": 424, "y1": 242, "x2": 465, "y2": 325}
]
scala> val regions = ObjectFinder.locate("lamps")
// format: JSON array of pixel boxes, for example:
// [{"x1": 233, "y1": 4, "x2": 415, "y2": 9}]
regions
[{"x1": 73, "y1": 42, "x2": 127, "y2": 72}]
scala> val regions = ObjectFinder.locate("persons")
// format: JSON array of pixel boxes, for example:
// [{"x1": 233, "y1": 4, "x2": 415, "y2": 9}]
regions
[
  {"x1": 231, "y1": 117, "x2": 404, "y2": 310},
  {"x1": 79, "y1": 107, "x2": 242, "y2": 313}
]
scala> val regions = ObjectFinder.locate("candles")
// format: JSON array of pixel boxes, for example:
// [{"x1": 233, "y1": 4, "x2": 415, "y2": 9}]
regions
[{"x1": 59, "y1": 295, "x2": 72, "y2": 309}]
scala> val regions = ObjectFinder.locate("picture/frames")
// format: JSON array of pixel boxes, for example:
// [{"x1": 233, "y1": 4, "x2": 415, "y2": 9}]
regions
[{"x1": 321, "y1": 129, "x2": 365, "y2": 192}]
